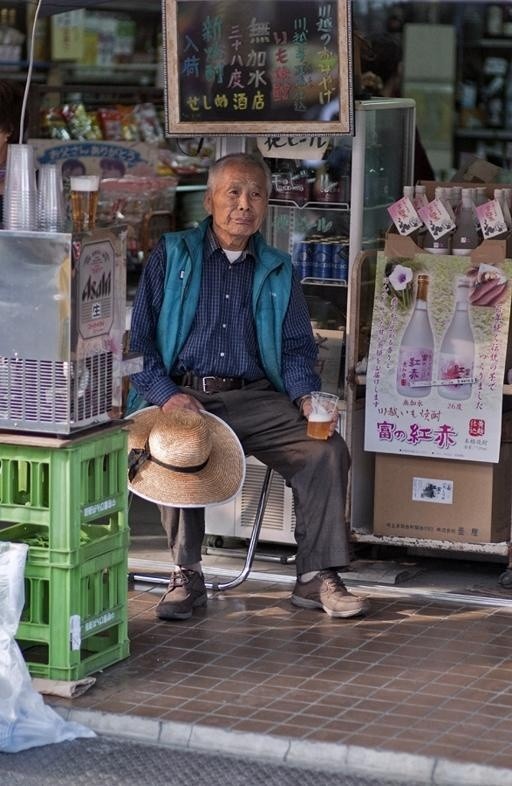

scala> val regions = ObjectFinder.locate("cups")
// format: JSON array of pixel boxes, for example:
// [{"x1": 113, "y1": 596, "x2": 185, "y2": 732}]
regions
[
  {"x1": 69, "y1": 174, "x2": 100, "y2": 227},
  {"x1": 304, "y1": 390, "x2": 340, "y2": 441},
  {"x1": 3, "y1": 142, "x2": 68, "y2": 233}
]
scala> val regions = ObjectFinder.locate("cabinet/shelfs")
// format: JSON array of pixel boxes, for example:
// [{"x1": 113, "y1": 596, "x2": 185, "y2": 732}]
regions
[{"x1": 335, "y1": 249, "x2": 511, "y2": 591}]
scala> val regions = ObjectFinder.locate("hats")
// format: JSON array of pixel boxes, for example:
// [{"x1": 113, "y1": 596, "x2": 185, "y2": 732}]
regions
[{"x1": 114, "y1": 404, "x2": 247, "y2": 510}]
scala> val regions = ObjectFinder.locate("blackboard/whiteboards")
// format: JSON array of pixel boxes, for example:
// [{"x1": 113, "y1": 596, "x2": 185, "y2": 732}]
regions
[{"x1": 162, "y1": 0, "x2": 356, "y2": 138}]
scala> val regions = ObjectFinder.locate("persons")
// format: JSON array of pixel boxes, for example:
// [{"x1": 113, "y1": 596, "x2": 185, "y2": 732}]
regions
[{"x1": 126, "y1": 152, "x2": 372, "y2": 621}]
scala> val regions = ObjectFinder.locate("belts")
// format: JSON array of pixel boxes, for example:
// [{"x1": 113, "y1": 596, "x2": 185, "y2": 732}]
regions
[{"x1": 169, "y1": 369, "x2": 244, "y2": 394}]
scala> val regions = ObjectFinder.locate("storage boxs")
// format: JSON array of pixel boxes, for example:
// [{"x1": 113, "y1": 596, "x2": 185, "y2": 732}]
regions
[{"x1": 371, "y1": 410, "x2": 512, "y2": 545}]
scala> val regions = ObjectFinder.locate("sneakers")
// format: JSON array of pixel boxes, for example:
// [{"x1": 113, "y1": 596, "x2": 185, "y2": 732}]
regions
[
  {"x1": 290, "y1": 569, "x2": 370, "y2": 619},
  {"x1": 156, "y1": 569, "x2": 208, "y2": 619}
]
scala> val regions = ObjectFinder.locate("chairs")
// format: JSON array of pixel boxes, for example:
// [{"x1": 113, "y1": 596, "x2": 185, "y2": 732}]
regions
[{"x1": 119, "y1": 445, "x2": 296, "y2": 593}]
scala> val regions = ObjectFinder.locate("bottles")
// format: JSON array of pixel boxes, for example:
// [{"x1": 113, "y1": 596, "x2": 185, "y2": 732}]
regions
[
  {"x1": 402, "y1": 185, "x2": 511, "y2": 257},
  {"x1": 437, "y1": 279, "x2": 476, "y2": 401},
  {"x1": 396, "y1": 274, "x2": 435, "y2": 399}
]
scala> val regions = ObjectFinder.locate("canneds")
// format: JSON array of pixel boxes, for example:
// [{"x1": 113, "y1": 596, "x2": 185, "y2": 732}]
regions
[{"x1": 295, "y1": 235, "x2": 350, "y2": 284}]
